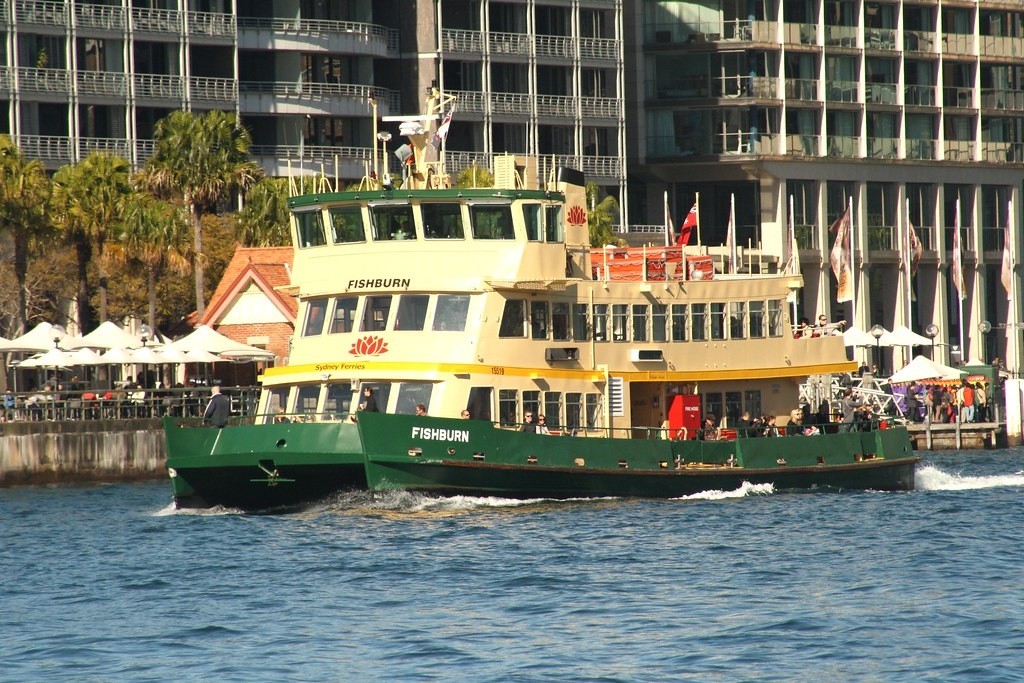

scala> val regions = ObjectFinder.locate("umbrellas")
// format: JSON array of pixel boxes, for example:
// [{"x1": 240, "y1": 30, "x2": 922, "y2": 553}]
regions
[
  {"x1": 878, "y1": 356, "x2": 969, "y2": 384},
  {"x1": 834, "y1": 325, "x2": 939, "y2": 366},
  {"x1": 0, "y1": 321, "x2": 273, "y2": 389}
]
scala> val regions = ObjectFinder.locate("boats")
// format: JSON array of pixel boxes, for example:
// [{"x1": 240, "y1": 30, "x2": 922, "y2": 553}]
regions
[{"x1": 163, "y1": 80, "x2": 923, "y2": 512}]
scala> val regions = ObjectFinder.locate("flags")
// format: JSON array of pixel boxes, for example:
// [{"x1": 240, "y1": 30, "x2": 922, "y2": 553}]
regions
[
  {"x1": 829, "y1": 204, "x2": 1011, "y2": 301},
  {"x1": 435, "y1": 109, "x2": 452, "y2": 141},
  {"x1": 665, "y1": 201, "x2": 800, "y2": 317}
]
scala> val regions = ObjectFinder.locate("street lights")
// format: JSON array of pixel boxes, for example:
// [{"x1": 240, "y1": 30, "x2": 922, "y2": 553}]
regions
[
  {"x1": 978, "y1": 320, "x2": 991, "y2": 366},
  {"x1": 925, "y1": 324, "x2": 939, "y2": 362},
  {"x1": 871, "y1": 324, "x2": 884, "y2": 384}
]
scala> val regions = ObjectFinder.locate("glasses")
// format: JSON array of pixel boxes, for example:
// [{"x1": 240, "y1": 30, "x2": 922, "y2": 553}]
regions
[{"x1": 822, "y1": 318, "x2": 827, "y2": 321}]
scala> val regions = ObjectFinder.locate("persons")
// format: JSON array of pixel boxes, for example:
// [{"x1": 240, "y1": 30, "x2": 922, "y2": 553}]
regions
[
  {"x1": 28, "y1": 377, "x2": 79, "y2": 421},
  {"x1": 417, "y1": 404, "x2": 428, "y2": 416},
  {"x1": 794, "y1": 315, "x2": 846, "y2": 339},
  {"x1": 520, "y1": 412, "x2": 548, "y2": 434},
  {"x1": 114, "y1": 369, "x2": 200, "y2": 417},
  {"x1": 361, "y1": 387, "x2": 378, "y2": 412},
  {"x1": 884, "y1": 378, "x2": 994, "y2": 423},
  {"x1": 699, "y1": 400, "x2": 829, "y2": 442},
  {"x1": 202, "y1": 387, "x2": 229, "y2": 428},
  {"x1": 3, "y1": 389, "x2": 14, "y2": 419},
  {"x1": 274, "y1": 408, "x2": 305, "y2": 423},
  {"x1": 389, "y1": 217, "x2": 415, "y2": 241},
  {"x1": 461, "y1": 410, "x2": 470, "y2": 418},
  {"x1": 839, "y1": 362, "x2": 879, "y2": 433},
  {"x1": 992, "y1": 357, "x2": 999, "y2": 367}
]
